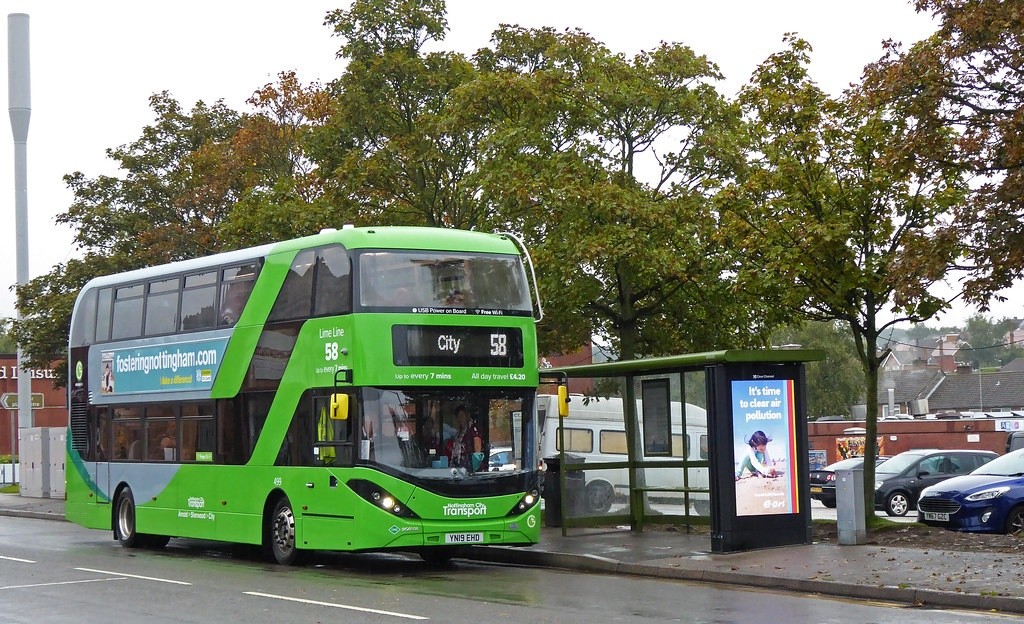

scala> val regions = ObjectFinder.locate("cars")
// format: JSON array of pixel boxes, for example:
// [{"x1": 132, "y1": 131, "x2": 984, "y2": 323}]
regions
[
  {"x1": 808, "y1": 452, "x2": 898, "y2": 508},
  {"x1": 915, "y1": 446, "x2": 1024, "y2": 536}
]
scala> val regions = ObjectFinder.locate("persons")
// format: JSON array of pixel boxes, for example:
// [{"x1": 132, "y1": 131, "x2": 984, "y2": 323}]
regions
[
  {"x1": 413, "y1": 406, "x2": 485, "y2": 472},
  {"x1": 101, "y1": 415, "x2": 193, "y2": 462},
  {"x1": 104, "y1": 364, "x2": 113, "y2": 393},
  {"x1": 735, "y1": 431, "x2": 777, "y2": 481},
  {"x1": 222, "y1": 287, "x2": 246, "y2": 325},
  {"x1": 347, "y1": 414, "x2": 388, "y2": 449}
]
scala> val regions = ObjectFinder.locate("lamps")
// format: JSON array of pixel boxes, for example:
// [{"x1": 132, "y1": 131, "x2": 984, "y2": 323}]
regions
[{"x1": 965, "y1": 425, "x2": 973, "y2": 430}]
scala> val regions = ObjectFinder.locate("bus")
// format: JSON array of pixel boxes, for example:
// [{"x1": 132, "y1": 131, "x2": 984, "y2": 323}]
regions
[{"x1": 63, "y1": 222, "x2": 573, "y2": 568}]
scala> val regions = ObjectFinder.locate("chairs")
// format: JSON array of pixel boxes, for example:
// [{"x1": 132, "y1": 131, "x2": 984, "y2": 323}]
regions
[
  {"x1": 129, "y1": 440, "x2": 142, "y2": 459},
  {"x1": 318, "y1": 406, "x2": 336, "y2": 460}
]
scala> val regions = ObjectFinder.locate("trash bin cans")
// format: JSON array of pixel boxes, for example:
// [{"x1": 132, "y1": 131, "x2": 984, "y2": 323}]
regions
[{"x1": 543, "y1": 453, "x2": 587, "y2": 528}]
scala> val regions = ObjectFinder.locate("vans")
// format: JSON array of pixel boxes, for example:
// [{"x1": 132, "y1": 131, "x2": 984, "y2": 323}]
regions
[
  {"x1": 1006, "y1": 431, "x2": 1024, "y2": 453},
  {"x1": 874, "y1": 447, "x2": 1000, "y2": 517},
  {"x1": 534, "y1": 392, "x2": 711, "y2": 516}
]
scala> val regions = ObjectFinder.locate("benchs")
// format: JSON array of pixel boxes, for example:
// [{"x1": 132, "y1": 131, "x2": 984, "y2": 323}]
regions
[{"x1": 633, "y1": 486, "x2": 709, "y2": 492}]
